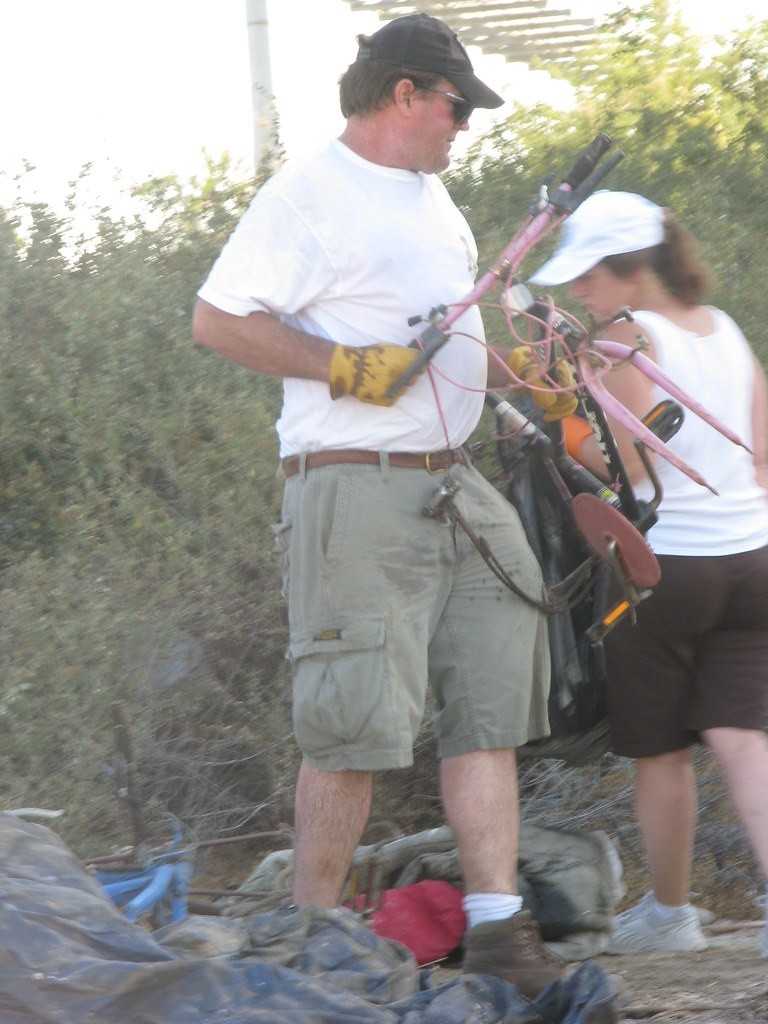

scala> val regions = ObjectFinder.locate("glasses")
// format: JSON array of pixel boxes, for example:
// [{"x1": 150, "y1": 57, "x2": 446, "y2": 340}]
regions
[{"x1": 414, "y1": 82, "x2": 475, "y2": 125}]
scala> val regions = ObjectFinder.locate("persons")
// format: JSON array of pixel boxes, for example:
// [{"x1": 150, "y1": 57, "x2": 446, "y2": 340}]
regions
[
  {"x1": 529, "y1": 188, "x2": 768, "y2": 960},
  {"x1": 192, "y1": 12, "x2": 625, "y2": 1007}
]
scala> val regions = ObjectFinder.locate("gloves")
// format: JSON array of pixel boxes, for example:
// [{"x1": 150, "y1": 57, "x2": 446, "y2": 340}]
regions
[
  {"x1": 328, "y1": 339, "x2": 426, "y2": 407},
  {"x1": 560, "y1": 410, "x2": 593, "y2": 454},
  {"x1": 506, "y1": 346, "x2": 580, "y2": 421}
]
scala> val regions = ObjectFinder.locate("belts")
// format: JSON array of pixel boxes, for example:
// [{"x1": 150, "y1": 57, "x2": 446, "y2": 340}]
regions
[{"x1": 281, "y1": 443, "x2": 474, "y2": 478}]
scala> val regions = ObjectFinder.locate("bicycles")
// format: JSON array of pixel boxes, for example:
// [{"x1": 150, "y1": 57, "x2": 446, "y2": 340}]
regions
[{"x1": 382, "y1": 134, "x2": 752, "y2": 714}]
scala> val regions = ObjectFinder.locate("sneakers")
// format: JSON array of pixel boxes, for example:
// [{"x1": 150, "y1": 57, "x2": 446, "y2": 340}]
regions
[
  {"x1": 465, "y1": 909, "x2": 627, "y2": 1001},
  {"x1": 757, "y1": 892, "x2": 768, "y2": 958},
  {"x1": 601, "y1": 890, "x2": 708, "y2": 955}
]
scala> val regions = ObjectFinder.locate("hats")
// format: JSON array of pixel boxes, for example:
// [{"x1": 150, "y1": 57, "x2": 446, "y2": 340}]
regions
[
  {"x1": 356, "y1": 13, "x2": 506, "y2": 109},
  {"x1": 526, "y1": 189, "x2": 670, "y2": 286}
]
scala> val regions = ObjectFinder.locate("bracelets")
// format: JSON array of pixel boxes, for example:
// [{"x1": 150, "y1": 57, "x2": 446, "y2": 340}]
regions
[{"x1": 564, "y1": 414, "x2": 593, "y2": 460}]
